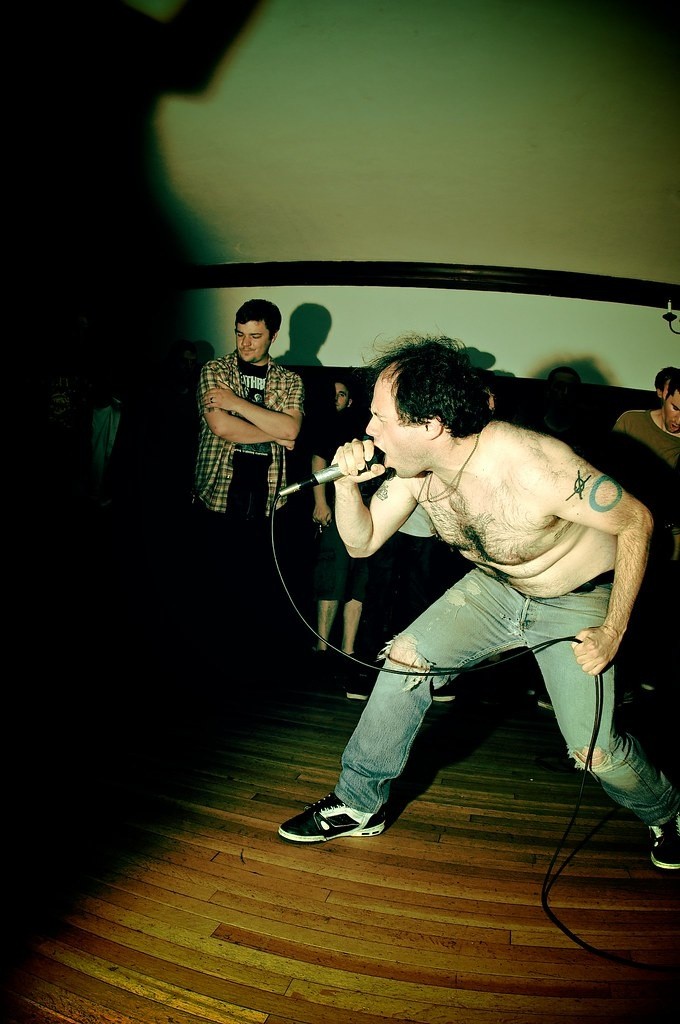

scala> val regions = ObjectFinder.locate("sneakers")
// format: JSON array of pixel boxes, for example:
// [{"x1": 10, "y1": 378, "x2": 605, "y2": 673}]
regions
[
  {"x1": 648, "y1": 810, "x2": 680, "y2": 869},
  {"x1": 277, "y1": 792, "x2": 385, "y2": 845}
]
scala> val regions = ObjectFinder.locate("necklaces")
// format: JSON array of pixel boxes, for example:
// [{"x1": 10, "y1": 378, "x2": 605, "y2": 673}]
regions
[{"x1": 417, "y1": 434, "x2": 480, "y2": 505}]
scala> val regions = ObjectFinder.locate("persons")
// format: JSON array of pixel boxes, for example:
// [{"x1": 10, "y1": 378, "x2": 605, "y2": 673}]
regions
[
  {"x1": 333, "y1": 381, "x2": 352, "y2": 412},
  {"x1": 654, "y1": 367, "x2": 678, "y2": 400},
  {"x1": 195, "y1": 298, "x2": 305, "y2": 717},
  {"x1": 616, "y1": 375, "x2": 680, "y2": 475},
  {"x1": 313, "y1": 424, "x2": 376, "y2": 681},
  {"x1": 546, "y1": 366, "x2": 581, "y2": 384},
  {"x1": 274, "y1": 332, "x2": 680, "y2": 872}
]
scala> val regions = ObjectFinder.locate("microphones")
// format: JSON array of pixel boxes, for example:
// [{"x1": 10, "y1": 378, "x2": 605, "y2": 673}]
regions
[{"x1": 279, "y1": 452, "x2": 378, "y2": 498}]
